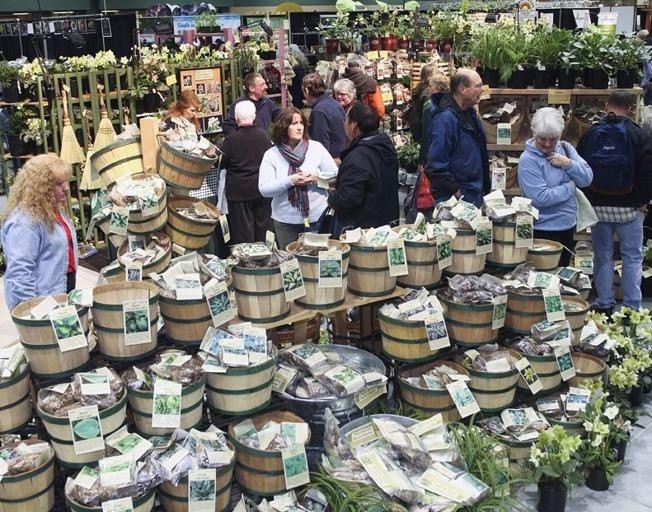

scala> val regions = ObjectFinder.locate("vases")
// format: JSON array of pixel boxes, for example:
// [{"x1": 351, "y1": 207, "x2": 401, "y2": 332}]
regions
[
  {"x1": 534, "y1": 476, "x2": 568, "y2": 511},
  {"x1": 2, "y1": 71, "x2": 168, "y2": 157},
  {"x1": 583, "y1": 466, "x2": 611, "y2": 491},
  {"x1": 625, "y1": 381, "x2": 644, "y2": 407}
]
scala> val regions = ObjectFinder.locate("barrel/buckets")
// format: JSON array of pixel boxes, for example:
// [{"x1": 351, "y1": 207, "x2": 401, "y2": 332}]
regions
[
  {"x1": 525, "y1": 103, "x2": 570, "y2": 132},
  {"x1": 569, "y1": 103, "x2": 607, "y2": 132},
  {"x1": 0, "y1": 139, "x2": 609, "y2": 512},
  {"x1": 477, "y1": 98, "x2": 525, "y2": 145}
]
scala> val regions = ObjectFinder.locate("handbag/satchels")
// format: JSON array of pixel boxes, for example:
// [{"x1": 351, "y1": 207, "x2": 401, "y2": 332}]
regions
[
  {"x1": 559, "y1": 140, "x2": 600, "y2": 237},
  {"x1": 403, "y1": 169, "x2": 436, "y2": 224}
]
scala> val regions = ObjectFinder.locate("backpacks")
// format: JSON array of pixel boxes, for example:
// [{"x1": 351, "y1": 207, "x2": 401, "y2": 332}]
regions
[{"x1": 585, "y1": 112, "x2": 635, "y2": 198}]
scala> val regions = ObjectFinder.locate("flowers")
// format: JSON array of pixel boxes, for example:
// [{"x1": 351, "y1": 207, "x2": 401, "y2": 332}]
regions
[
  {"x1": 602, "y1": 347, "x2": 652, "y2": 391},
  {"x1": 526, "y1": 424, "x2": 587, "y2": 493},
  {"x1": 570, "y1": 397, "x2": 633, "y2": 484}
]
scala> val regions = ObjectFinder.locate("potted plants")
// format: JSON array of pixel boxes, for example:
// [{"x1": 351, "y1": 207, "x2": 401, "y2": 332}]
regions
[
  {"x1": 196, "y1": 10, "x2": 221, "y2": 33},
  {"x1": 319, "y1": 0, "x2": 649, "y2": 89}
]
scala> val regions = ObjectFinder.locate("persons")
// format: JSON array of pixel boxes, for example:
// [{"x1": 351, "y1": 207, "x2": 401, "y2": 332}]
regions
[
  {"x1": 576, "y1": 89, "x2": 651, "y2": 318},
  {"x1": 1, "y1": 151, "x2": 84, "y2": 310},
  {"x1": 515, "y1": 105, "x2": 596, "y2": 268},
  {"x1": 161, "y1": 89, "x2": 204, "y2": 148},
  {"x1": 213, "y1": 41, "x2": 228, "y2": 58},
  {"x1": 286, "y1": 43, "x2": 309, "y2": 105}
]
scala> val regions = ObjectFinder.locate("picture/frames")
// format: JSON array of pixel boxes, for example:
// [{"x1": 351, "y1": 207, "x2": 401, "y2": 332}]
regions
[{"x1": 180, "y1": 66, "x2": 225, "y2": 121}]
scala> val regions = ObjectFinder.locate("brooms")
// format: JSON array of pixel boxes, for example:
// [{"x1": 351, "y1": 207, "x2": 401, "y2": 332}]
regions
[
  {"x1": 93, "y1": 83, "x2": 117, "y2": 150},
  {"x1": 58, "y1": 91, "x2": 86, "y2": 165}
]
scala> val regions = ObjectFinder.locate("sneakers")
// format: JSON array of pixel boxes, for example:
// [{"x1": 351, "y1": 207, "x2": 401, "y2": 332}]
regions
[
  {"x1": 589, "y1": 303, "x2": 615, "y2": 324},
  {"x1": 622, "y1": 309, "x2": 652, "y2": 325}
]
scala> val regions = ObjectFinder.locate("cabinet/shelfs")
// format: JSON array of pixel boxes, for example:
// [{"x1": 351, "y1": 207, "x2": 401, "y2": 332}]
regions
[
  {"x1": 473, "y1": 88, "x2": 645, "y2": 204},
  {"x1": 1, "y1": 54, "x2": 240, "y2": 252}
]
